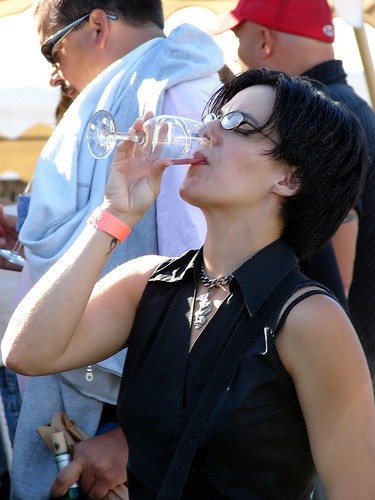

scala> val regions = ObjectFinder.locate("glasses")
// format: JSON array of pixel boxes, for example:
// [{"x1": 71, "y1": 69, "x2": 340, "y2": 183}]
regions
[
  {"x1": 200, "y1": 111, "x2": 289, "y2": 163},
  {"x1": 39, "y1": 11, "x2": 118, "y2": 63}
]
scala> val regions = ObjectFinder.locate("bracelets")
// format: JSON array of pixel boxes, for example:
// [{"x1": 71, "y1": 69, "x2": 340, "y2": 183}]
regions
[{"x1": 86, "y1": 208, "x2": 131, "y2": 243}]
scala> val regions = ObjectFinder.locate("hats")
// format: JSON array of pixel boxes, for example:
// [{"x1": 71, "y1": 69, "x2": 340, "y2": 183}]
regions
[{"x1": 205, "y1": 1, "x2": 337, "y2": 44}]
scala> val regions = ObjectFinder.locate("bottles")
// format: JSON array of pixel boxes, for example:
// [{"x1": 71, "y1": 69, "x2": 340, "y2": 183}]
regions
[{"x1": 49, "y1": 431, "x2": 81, "y2": 500}]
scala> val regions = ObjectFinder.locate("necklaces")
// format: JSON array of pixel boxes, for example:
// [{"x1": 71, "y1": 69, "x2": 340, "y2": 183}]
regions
[{"x1": 193, "y1": 265, "x2": 233, "y2": 330}]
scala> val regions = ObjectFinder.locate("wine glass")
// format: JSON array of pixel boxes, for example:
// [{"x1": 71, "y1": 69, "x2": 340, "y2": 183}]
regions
[
  {"x1": 85, "y1": 109, "x2": 212, "y2": 165},
  {"x1": 0, "y1": 175, "x2": 36, "y2": 266}
]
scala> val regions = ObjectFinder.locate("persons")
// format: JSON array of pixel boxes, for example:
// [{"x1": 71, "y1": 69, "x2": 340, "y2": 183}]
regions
[
  {"x1": 0, "y1": 68, "x2": 375, "y2": 500},
  {"x1": 10, "y1": 1, "x2": 229, "y2": 500},
  {"x1": 214, "y1": 0, "x2": 375, "y2": 389}
]
